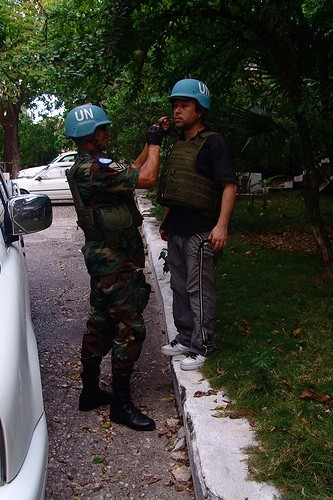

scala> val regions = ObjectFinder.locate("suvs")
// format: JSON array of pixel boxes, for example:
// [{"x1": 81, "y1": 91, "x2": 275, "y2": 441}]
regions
[
  {"x1": 0, "y1": 166, "x2": 53, "y2": 500},
  {"x1": 17, "y1": 151, "x2": 78, "y2": 177}
]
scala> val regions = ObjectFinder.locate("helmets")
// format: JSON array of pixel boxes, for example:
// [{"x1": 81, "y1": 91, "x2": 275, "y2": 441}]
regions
[
  {"x1": 168, "y1": 78, "x2": 210, "y2": 111},
  {"x1": 65, "y1": 105, "x2": 111, "y2": 138}
]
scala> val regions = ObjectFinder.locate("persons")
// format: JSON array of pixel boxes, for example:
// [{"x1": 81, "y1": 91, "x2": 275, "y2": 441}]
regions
[
  {"x1": 64, "y1": 104, "x2": 170, "y2": 431},
  {"x1": 157, "y1": 79, "x2": 239, "y2": 371}
]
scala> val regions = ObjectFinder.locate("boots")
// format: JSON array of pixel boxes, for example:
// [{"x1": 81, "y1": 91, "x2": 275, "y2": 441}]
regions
[
  {"x1": 79, "y1": 370, "x2": 112, "y2": 412},
  {"x1": 110, "y1": 375, "x2": 156, "y2": 431}
]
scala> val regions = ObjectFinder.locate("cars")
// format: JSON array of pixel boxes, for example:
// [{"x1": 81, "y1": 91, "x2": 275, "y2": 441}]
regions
[{"x1": 10, "y1": 161, "x2": 76, "y2": 204}]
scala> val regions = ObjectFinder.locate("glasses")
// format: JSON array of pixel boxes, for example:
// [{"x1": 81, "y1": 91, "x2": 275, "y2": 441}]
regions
[{"x1": 98, "y1": 126, "x2": 110, "y2": 131}]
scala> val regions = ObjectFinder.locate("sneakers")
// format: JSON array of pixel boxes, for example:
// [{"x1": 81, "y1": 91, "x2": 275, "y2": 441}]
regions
[
  {"x1": 181, "y1": 351, "x2": 207, "y2": 371},
  {"x1": 160, "y1": 340, "x2": 193, "y2": 355}
]
